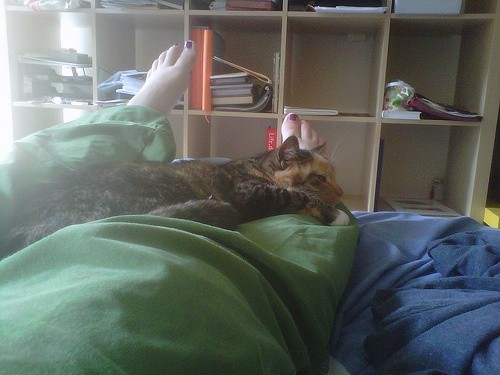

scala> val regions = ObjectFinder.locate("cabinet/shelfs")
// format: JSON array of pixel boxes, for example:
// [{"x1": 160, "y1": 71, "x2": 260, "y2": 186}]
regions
[{"x1": 0, "y1": 0, "x2": 499, "y2": 226}]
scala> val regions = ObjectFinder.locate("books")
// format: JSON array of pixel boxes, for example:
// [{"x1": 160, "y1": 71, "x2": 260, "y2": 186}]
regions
[{"x1": 100, "y1": 0, "x2": 185, "y2": 10}]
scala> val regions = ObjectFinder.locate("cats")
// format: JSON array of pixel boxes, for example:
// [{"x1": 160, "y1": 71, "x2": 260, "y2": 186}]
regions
[{"x1": 0, "y1": 135, "x2": 351, "y2": 261}]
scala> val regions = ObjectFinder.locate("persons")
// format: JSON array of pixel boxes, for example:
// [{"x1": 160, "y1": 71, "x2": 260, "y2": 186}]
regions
[{"x1": 0, "y1": 39, "x2": 361, "y2": 375}]
